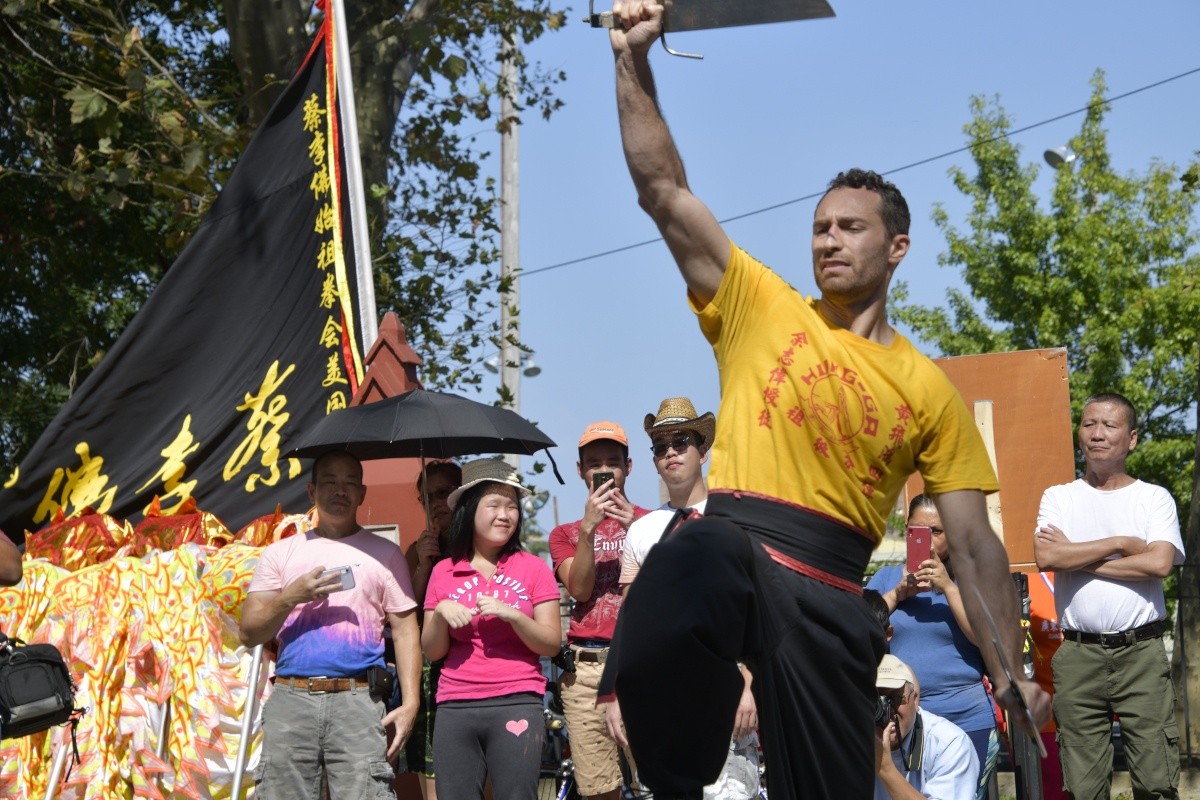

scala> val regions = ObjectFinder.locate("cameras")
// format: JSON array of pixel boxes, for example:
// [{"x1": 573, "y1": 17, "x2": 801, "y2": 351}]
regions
[{"x1": 875, "y1": 694, "x2": 894, "y2": 729}]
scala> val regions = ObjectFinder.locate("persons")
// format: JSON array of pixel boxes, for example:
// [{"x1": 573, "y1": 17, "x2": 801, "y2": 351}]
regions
[
  {"x1": 240, "y1": 393, "x2": 1188, "y2": 800},
  {"x1": 0, "y1": 506, "x2": 23, "y2": 587},
  {"x1": 607, "y1": 1, "x2": 1052, "y2": 800}
]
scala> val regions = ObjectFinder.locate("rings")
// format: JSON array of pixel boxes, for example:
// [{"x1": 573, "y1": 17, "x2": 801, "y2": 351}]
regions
[{"x1": 930, "y1": 568, "x2": 933, "y2": 575}]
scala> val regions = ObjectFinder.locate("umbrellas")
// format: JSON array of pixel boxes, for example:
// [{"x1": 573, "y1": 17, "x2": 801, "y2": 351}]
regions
[{"x1": 277, "y1": 388, "x2": 558, "y2": 555}]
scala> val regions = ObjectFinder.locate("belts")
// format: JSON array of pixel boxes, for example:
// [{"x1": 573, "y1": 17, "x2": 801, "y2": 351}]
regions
[
  {"x1": 275, "y1": 675, "x2": 368, "y2": 695},
  {"x1": 1064, "y1": 620, "x2": 1160, "y2": 648},
  {"x1": 573, "y1": 650, "x2": 612, "y2": 662}
]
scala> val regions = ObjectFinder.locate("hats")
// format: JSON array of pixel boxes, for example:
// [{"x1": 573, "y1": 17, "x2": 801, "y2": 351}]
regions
[
  {"x1": 876, "y1": 654, "x2": 914, "y2": 688},
  {"x1": 644, "y1": 398, "x2": 716, "y2": 450},
  {"x1": 578, "y1": 421, "x2": 629, "y2": 447},
  {"x1": 447, "y1": 458, "x2": 533, "y2": 512}
]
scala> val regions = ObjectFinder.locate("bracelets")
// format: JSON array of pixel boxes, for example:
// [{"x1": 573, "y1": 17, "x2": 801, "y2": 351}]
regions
[{"x1": 415, "y1": 565, "x2": 430, "y2": 577}]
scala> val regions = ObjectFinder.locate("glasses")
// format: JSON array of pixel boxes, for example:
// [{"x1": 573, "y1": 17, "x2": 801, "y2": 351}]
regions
[
  {"x1": 649, "y1": 437, "x2": 690, "y2": 457},
  {"x1": 417, "y1": 487, "x2": 449, "y2": 505}
]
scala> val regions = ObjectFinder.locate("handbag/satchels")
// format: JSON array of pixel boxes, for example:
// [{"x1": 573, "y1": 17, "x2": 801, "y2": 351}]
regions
[{"x1": 0, "y1": 637, "x2": 91, "y2": 783}]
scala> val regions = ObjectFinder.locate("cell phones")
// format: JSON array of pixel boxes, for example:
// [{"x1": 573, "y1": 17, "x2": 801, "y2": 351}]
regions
[
  {"x1": 592, "y1": 472, "x2": 616, "y2": 496},
  {"x1": 906, "y1": 526, "x2": 932, "y2": 574},
  {"x1": 317, "y1": 566, "x2": 356, "y2": 593}
]
